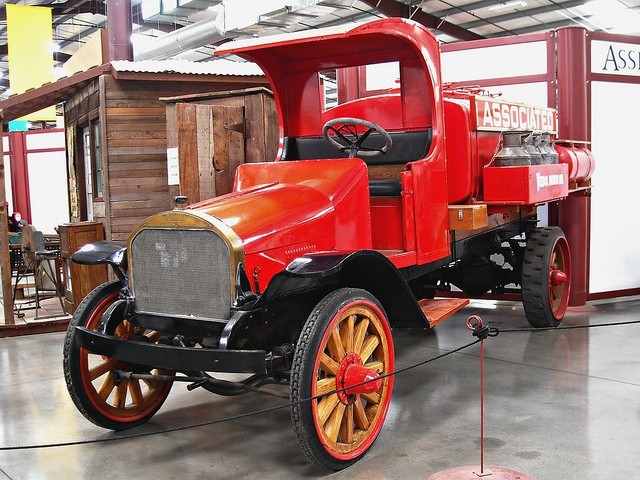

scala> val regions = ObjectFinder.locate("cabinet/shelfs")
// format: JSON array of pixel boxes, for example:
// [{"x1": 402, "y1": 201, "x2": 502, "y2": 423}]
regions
[{"x1": 58, "y1": 220, "x2": 108, "y2": 315}]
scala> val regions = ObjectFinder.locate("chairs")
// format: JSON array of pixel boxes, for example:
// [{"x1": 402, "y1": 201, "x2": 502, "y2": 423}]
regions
[{"x1": 13, "y1": 231, "x2": 68, "y2": 320}]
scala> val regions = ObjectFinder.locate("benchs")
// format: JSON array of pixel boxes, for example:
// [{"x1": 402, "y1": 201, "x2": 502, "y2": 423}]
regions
[
  {"x1": 279, "y1": 128, "x2": 432, "y2": 195},
  {"x1": 7, "y1": 231, "x2": 61, "y2": 289}
]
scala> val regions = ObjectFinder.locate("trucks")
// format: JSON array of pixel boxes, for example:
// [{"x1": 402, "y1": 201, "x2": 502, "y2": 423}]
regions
[{"x1": 64, "y1": 19, "x2": 598, "y2": 473}]
